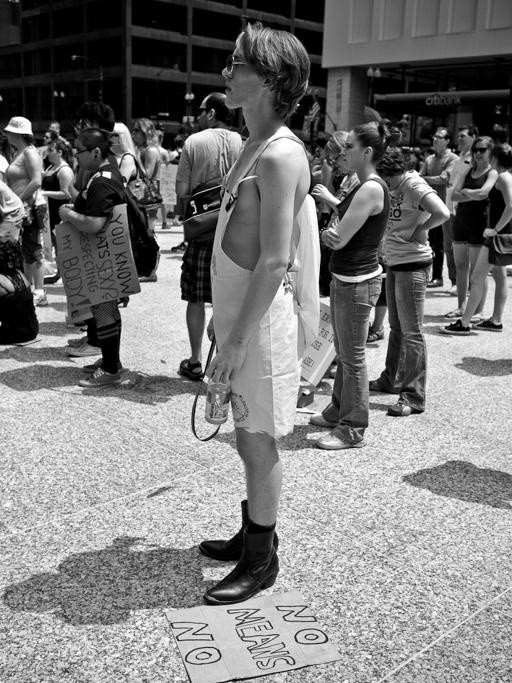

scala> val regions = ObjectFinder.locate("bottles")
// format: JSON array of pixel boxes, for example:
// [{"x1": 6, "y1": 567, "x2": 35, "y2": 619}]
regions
[{"x1": 205, "y1": 368, "x2": 232, "y2": 423}]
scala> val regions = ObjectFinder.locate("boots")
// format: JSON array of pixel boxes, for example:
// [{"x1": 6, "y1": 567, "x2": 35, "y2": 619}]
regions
[
  {"x1": 198, "y1": 499, "x2": 279, "y2": 561},
  {"x1": 202, "y1": 516, "x2": 280, "y2": 605}
]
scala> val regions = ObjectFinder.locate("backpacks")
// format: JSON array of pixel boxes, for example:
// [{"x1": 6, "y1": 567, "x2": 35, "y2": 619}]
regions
[{"x1": 82, "y1": 171, "x2": 161, "y2": 278}]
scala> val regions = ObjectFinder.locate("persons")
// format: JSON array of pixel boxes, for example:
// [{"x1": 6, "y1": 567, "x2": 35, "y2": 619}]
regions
[
  {"x1": 296, "y1": 119, "x2": 512, "y2": 449},
  {"x1": 196, "y1": 20, "x2": 312, "y2": 607},
  {"x1": 1, "y1": 92, "x2": 249, "y2": 386}
]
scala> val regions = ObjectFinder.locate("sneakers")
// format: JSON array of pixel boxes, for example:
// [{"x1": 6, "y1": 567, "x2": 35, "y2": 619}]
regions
[
  {"x1": 32, "y1": 289, "x2": 205, "y2": 386},
  {"x1": 171, "y1": 242, "x2": 188, "y2": 252},
  {"x1": 310, "y1": 278, "x2": 503, "y2": 451}
]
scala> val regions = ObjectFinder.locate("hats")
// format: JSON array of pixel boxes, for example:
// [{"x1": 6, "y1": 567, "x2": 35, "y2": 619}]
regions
[
  {"x1": 3, "y1": 116, "x2": 34, "y2": 137},
  {"x1": 199, "y1": 108, "x2": 210, "y2": 116}
]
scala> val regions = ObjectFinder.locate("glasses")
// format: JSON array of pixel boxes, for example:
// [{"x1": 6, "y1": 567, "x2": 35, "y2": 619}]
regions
[
  {"x1": 226, "y1": 55, "x2": 248, "y2": 72},
  {"x1": 473, "y1": 147, "x2": 488, "y2": 154},
  {"x1": 433, "y1": 135, "x2": 444, "y2": 139}
]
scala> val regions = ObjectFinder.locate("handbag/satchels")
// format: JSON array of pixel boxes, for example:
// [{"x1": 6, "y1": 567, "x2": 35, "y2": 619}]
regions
[{"x1": 118, "y1": 176, "x2": 163, "y2": 212}]
